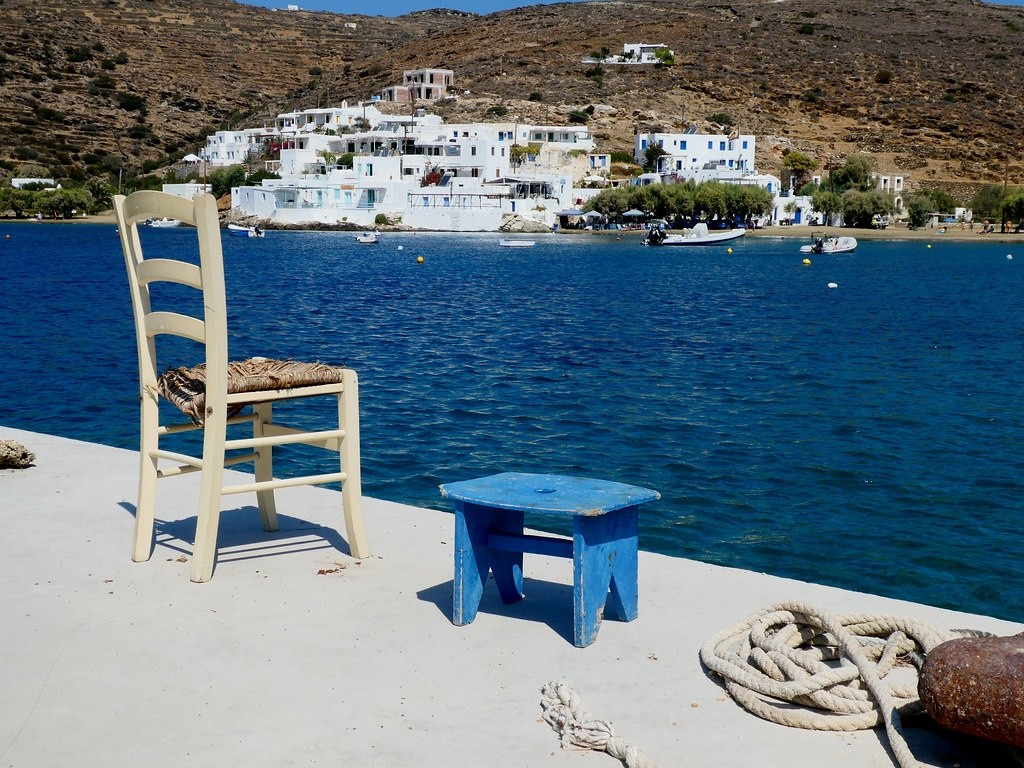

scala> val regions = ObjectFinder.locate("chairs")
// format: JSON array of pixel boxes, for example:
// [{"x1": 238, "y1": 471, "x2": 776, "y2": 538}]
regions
[{"x1": 109, "y1": 191, "x2": 370, "y2": 583}]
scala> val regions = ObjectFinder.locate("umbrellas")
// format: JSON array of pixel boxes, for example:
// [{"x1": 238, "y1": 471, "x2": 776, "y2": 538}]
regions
[
  {"x1": 622, "y1": 209, "x2": 645, "y2": 229},
  {"x1": 944, "y1": 217, "x2": 957, "y2": 231},
  {"x1": 559, "y1": 207, "x2": 601, "y2": 229}
]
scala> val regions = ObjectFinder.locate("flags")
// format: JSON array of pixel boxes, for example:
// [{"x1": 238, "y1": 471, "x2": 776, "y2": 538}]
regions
[{"x1": 727, "y1": 126, "x2": 740, "y2": 141}]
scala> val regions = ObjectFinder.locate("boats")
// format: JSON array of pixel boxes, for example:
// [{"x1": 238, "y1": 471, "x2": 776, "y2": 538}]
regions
[
  {"x1": 799, "y1": 236, "x2": 858, "y2": 254},
  {"x1": 356, "y1": 232, "x2": 378, "y2": 243},
  {"x1": 640, "y1": 222, "x2": 746, "y2": 247},
  {"x1": 148, "y1": 216, "x2": 181, "y2": 228},
  {"x1": 227, "y1": 223, "x2": 265, "y2": 238},
  {"x1": 500, "y1": 237, "x2": 536, "y2": 247}
]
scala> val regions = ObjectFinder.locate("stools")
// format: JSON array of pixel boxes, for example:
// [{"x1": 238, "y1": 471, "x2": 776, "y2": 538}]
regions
[{"x1": 437, "y1": 472, "x2": 661, "y2": 649}]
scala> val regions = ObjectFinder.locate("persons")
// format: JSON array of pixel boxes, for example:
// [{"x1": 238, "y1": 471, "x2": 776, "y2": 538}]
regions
[
  {"x1": 941, "y1": 219, "x2": 1024, "y2": 234},
  {"x1": 574, "y1": 212, "x2": 610, "y2": 230},
  {"x1": 812, "y1": 237, "x2": 824, "y2": 255},
  {"x1": 747, "y1": 218, "x2": 790, "y2": 232}
]
